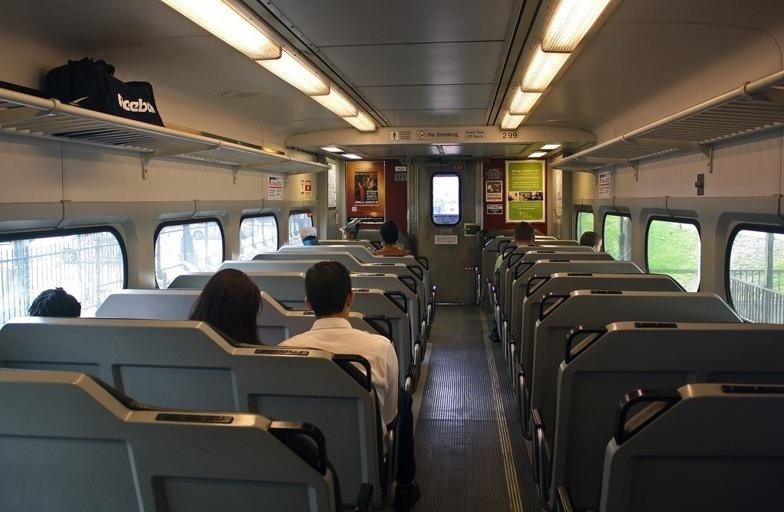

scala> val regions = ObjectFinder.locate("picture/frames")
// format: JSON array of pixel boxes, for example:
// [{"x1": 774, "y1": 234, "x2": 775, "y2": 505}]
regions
[
  {"x1": 345, "y1": 160, "x2": 386, "y2": 224},
  {"x1": 505, "y1": 160, "x2": 546, "y2": 223}
]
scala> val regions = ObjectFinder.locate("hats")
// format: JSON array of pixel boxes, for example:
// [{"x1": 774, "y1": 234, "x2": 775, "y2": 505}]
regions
[{"x1": 338, "y1": 224, "x2": 359, "y2": 234}]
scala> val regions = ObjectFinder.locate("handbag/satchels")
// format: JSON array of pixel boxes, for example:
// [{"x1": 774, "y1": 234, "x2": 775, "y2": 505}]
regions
[{"x1": 47, "y1": 57, "x2": 165, "y2": 144}]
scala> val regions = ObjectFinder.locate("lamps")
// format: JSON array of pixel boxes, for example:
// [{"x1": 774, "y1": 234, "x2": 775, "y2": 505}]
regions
[
  {"x1": 500, "y1": 0, "x2": 612, "y2": 131},
  {"x1": 159, "y1": 0, "x2": 377, "y2": 133}
]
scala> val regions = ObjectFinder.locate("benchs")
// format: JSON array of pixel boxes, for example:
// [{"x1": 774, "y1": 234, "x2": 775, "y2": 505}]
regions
[
  {"x1": 481, "y1": 235, "x2": 784, "y2": 511},
  {"x1": 0, "y1": 239, "x2": 437, "y2": 512}
]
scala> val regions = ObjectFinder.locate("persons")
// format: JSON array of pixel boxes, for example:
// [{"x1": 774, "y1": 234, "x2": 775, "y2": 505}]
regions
[
  {"x1": 274, "y1": 282, "x2": 422, "y2": 512},
  {"x1": 373, "y1": 218, "x2": 408, "y2": 256},
  {"x1": 487, "y1": 220, "x2": 535, "y2": 320},
  {"x1": 190, "y1": 267, "x2": 265, "y2": 346},
  {"x1": 27, "y1": 287, "x2": 82, "y2": 318},
  {"x1": 339, "y1": 217, "x2": 361, "y2": 240},
  {"x1": 300, "y1": 226, "x2": 319, "y2": 245},
  {"x1": 580, "y1": 231, "x2": 603, "y2": 253}
]
